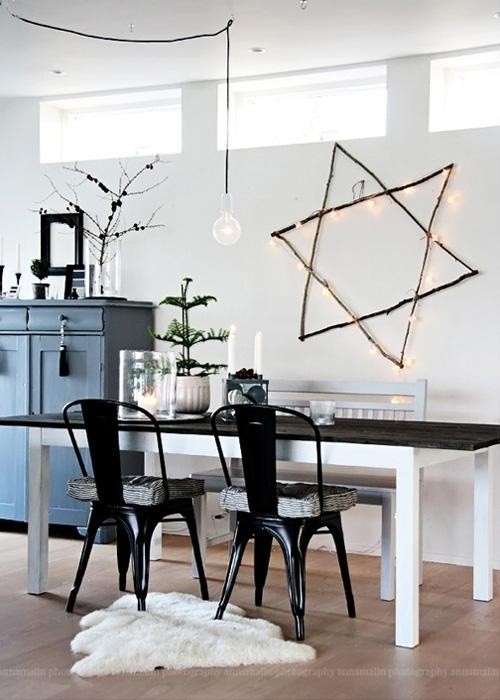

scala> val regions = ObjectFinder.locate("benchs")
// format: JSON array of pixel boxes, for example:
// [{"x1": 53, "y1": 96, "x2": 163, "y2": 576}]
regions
[{"x1": 189, "y1": 377, "x2": 427, "y2": 602}]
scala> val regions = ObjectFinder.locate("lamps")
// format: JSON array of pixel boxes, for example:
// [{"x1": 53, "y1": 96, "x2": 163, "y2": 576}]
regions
[{"x1": 211, "y1": 193, "x2": 241, "y2": 245}]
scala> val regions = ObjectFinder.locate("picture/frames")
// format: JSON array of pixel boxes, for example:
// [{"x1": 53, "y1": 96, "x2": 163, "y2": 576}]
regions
[{"x1": 40, "y1": 212, "x2": 83, "y2": 276}]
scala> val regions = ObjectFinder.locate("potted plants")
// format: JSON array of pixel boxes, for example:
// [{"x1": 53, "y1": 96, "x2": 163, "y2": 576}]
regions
[
  {"x1": 146, "y1": 276, "x2": 230, "y2": 413},
  {"x1": 30, "y1": 258, "x2": 52, "y2": 299},
  {"x1": 27, "y1": 152, "x2": 171, "y2": 298}
]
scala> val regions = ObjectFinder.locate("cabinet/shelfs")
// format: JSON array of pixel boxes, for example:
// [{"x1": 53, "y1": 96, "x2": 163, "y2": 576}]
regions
[{"x1": 0, "y1": 305, "x2": 159, "y2": 528}]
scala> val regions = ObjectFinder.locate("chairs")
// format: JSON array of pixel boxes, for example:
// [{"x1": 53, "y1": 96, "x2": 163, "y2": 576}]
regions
[
  {"x1": 209, "y1": 403, "x2": 358, "y2": 641},
  {"x1": 64, "y1": 398, "x2": 209, "y2": 613}
]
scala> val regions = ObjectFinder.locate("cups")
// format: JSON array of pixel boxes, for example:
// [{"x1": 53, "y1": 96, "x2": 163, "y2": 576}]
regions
[{"x1": 310, "y1": 400, "x2": 335, "y2": 426}]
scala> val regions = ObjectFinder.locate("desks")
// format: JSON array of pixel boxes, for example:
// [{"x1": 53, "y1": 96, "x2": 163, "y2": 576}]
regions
[{"x1": 0, "y1": 410, "x2": 499, "y2": 648}]
